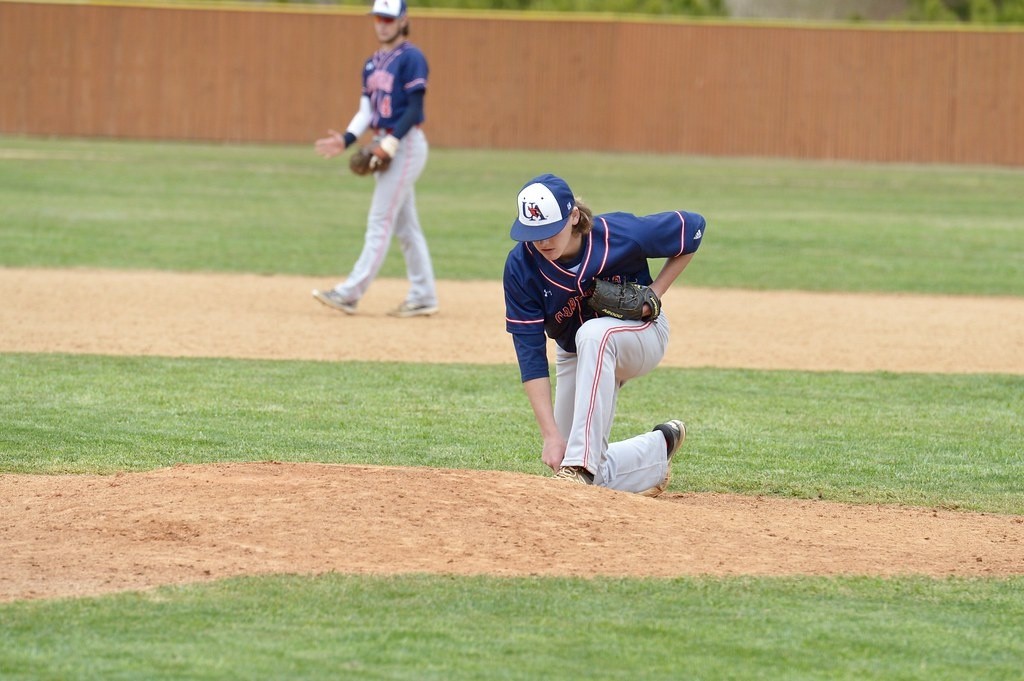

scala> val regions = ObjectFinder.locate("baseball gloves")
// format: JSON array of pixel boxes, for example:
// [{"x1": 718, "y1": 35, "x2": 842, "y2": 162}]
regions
[
  {"x1": 347, "y1": 133, "x2": 400, "y2": 175},
  {"x1": 587, "y1": 278, "x2": 662, "y2": 322}
]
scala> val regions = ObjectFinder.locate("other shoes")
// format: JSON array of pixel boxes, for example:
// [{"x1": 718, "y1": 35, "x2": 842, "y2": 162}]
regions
[
  {"x1": 387, "y1": 300, "x2": 439, "y2": 317},
  {"x1": 312, "y1": 289, "x2": 359, "y2": 315}
]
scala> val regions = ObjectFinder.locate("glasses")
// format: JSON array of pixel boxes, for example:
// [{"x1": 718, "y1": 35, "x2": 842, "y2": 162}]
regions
[{"x1": 372, "y1": 14, "x2": 399, "y2": 24}]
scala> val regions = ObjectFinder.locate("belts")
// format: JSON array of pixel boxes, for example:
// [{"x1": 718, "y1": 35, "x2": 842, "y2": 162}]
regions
[{"x1": 373, "y1": 123, "x2": 419, "y2": 136}]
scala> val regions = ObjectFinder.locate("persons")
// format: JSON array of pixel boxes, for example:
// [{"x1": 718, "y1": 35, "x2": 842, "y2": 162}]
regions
[
  {"x1": 312, "y1": 0, "x2": 439, "y2": 316},
  {"x1": 503, "y1": 174, "x2": 706, "y2": 498}
]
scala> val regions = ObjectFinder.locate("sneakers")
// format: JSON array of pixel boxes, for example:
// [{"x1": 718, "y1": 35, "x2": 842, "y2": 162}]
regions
[
  {"x1": 550, "y1": 465, "x2": 593, "y2": 486},
  {"x1": 639, "y1": 420, "x2": 686, "y2": 498}
]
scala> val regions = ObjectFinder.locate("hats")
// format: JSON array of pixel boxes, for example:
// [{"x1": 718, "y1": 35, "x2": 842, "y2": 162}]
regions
[
  {"x1": 510, "y1": 174, "x2": 575, "y2": 242},
  {"x1": 366, "y1": 0, "x2": 406, "y2": 18}
]
